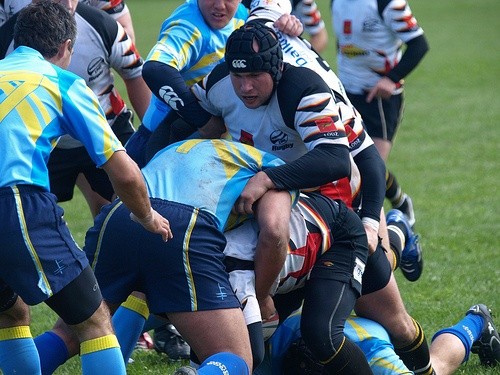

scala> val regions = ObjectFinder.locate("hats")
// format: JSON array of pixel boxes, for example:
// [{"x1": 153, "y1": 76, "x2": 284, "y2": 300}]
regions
[{"x1": 223, "y1": 20, "x2": 286, "y2": 100}]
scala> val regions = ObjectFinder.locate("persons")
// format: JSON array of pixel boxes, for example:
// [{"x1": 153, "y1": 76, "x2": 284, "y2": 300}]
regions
[
  {"x1": 252, "y1": 303, "x2": 500, "y2": 375},
  {"x1": 0, "y1": 0, "x2": 173, "y2": 375},
  {"x1": 0, "y1": 0, "x2": 151, "y2": 225},
  {"x1": 33, "y1": 138, "x2": 300, "y2": 375},
  {"x1": 190, "y1": 190, "x2": 371, "y2": 375},
  {"x1": 122, "y1": 0, "x2": 436, "y2": 375}
]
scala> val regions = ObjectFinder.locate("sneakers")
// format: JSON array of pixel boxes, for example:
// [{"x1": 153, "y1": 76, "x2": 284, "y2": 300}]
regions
[
  {"x1": 132, "y1": 330, "x2": 153, "y2": 356},
  {"x1": 154, "y1": 320, "x2": 197, "y2": 360},
  {"x1": 173, "y1": 366, "x2": 197, "y2": 375},
  {"x1": 465, "y1": 302, "x2": 499, "y2": 373},
  {"x1": 393, "y1": 192, "x2": 418, "y2": 233},
  {"x1": 386, "y1": 207, "x2": 426, "y2": 286}
]
